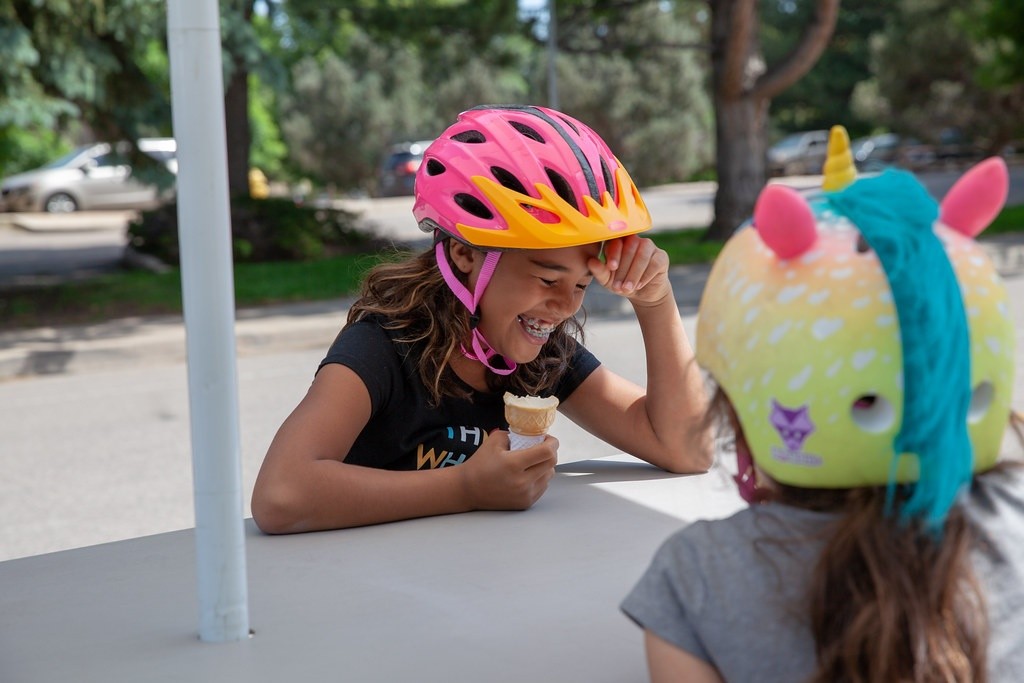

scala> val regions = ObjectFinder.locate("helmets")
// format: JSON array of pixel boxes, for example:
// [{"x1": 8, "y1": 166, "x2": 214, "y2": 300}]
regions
[
  {"x1": 694, "y1": 126, "x2": 1018, "y2": 533},
  {"x1": 412, "y1": 105, "x2": 654, "y2": 251}
]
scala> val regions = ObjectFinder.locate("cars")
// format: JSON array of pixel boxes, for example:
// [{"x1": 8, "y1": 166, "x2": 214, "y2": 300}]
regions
[
  {"x1": 378, "y1": 138, "x2": 436, "y2": 196},
  {"x1": 764, "y1": 128, "x2": 828, "y2": 177},
  {"x1": 849, "y1": 131, "x2": 937, "y2": 174},
  {"x1": 1, "y1": 131, "x2": 178, "y2": 212}
]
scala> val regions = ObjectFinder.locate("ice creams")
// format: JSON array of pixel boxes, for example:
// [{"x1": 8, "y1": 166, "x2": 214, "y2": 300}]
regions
[{"x1": 504, "y1": 390, "x2": 560, "y2": 451}]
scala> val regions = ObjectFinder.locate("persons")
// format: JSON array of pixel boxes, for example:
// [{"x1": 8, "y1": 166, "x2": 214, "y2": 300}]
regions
[
  {"x1": 250, "y1": 106, "x2": 719, "y2": 539},
  {"x1": 624, "y1": 124, "x2": 1024, "y2": 683}
]
